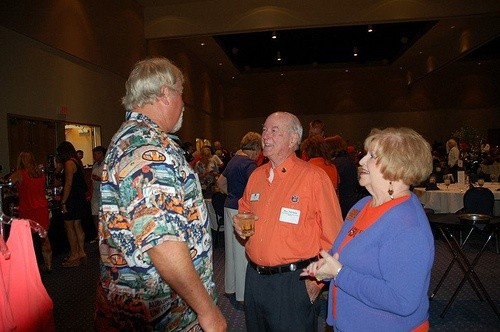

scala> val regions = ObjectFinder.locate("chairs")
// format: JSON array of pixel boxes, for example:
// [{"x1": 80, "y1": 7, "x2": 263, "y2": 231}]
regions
[
  {"x1": 454, "y1": 186, "x2": 500, "y2": 254},
  {"x1": 211, "y1": 191, "x2": 228, "y2": 248}
]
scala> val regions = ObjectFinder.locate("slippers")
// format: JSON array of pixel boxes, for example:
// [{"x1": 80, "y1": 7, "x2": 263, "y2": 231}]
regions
[{"x1": 89, "y1": 237, "x2": 99, "y2": 245}]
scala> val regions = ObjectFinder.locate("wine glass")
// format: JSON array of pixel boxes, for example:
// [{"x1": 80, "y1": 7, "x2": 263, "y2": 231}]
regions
[
  {"x1": 464, "y1": 175, "x2": 470, "y2": 189},
  {"x1": 444, "y1": 175, "x2": 451, "y2": 191},
  {"x1": 490, "y1": 174, "x2": 496, "y2": 190}
]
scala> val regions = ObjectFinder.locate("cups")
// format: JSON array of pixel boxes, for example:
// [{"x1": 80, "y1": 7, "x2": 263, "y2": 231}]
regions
[
  {"x1": 478, "y1": 179, "x2": 484, "y2": 187},
  {"x1": 236, "y1": 212, "x2": 255, "y2": 237}
]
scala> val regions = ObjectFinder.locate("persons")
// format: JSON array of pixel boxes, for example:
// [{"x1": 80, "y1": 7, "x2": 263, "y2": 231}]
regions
[
  {"x1": 300, "y1": 128, "x2": 434, "y2": 332},
  {"x1": 184, "y1": 132, "x2": 268, "y2": 302},
  {"x1": 94, "y1": 57, "x2": 227, "y2": 332},
  {"x1": 0, "y1": 141, "x2": 107, "y2": 272},
  {"x1": 232, "y1": 112, "x2": 344, "y2": 332},
  {"x1": 298, "y1": 133, "x2": 371, "y2": 220},
  {"x1": 430, "y1": 139, "x2": 491, "y2": 181}
]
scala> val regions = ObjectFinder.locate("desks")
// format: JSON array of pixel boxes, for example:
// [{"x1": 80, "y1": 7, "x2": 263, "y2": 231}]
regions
[
  {"x1": 201, "y1": 184, "x2": 225, "y2": 232},
  {"x1": 428, "y1": 211, "x2": 500, "y2": 319},
  {"x1": 413, "y1": 180, "x2": 500, "y2": 248}
]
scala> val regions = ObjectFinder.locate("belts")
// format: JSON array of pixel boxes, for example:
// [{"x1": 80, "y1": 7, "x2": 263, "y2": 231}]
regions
[{"x1": 248, "y1": 256, "x2": 318, "y2": 276}]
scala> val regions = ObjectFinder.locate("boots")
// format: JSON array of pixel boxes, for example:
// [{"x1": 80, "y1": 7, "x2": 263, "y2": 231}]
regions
[{"x1": 42, "y1": 249, "x2": 53, "y2": 272}]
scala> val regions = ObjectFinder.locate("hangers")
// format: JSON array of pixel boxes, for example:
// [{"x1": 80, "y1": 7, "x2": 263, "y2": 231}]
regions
[{"x1": 0, "y1": 187, "x2": 47, "y2": 238}]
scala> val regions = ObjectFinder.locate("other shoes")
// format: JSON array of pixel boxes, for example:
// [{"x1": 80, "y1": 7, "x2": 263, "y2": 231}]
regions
[
  {"x1": 79, "y1": 256, "x2": 88, "y2": 263},
  {"x1": 63, "y1": 257, "x2": 81, "y2": 267}
]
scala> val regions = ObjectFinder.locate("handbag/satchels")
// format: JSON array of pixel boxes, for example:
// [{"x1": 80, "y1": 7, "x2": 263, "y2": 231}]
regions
[
  {"x1": 214, "y1": 173, "x2": 228, "y2": 195},
  {"x1": 81, "y1": 168, "x2": 93, "y2": 201}
]
